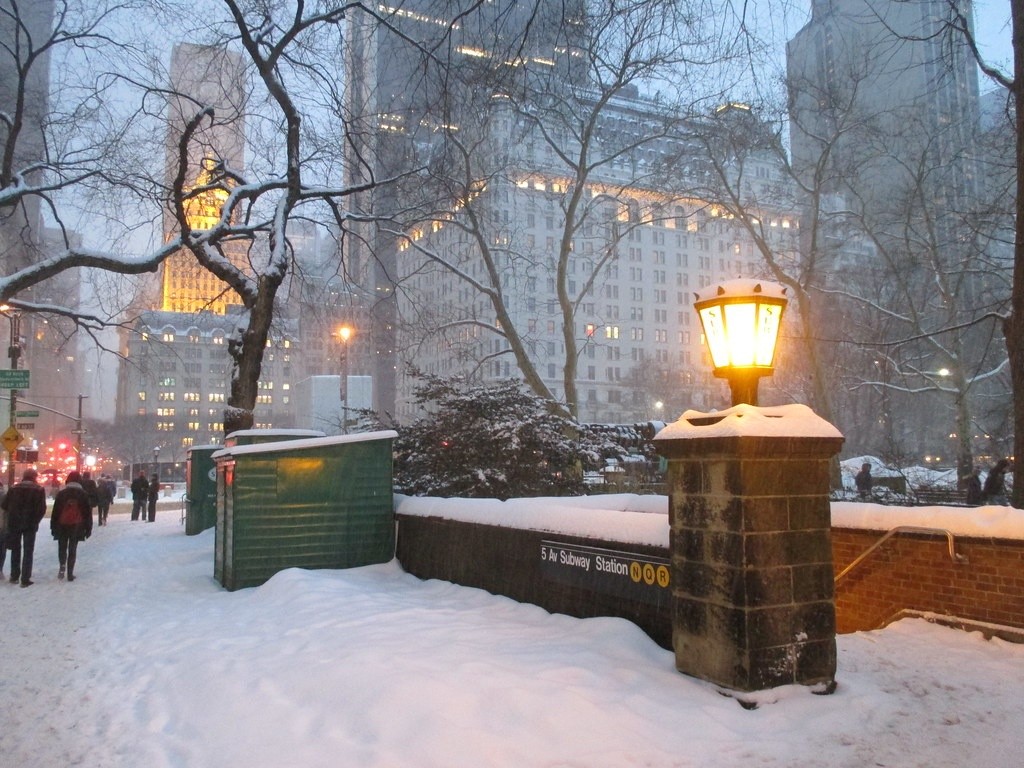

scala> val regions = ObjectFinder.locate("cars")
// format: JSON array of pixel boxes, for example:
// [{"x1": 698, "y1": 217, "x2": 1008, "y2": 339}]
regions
[{"x1": 0, "y1": 457, "x2": 96, "y2": 498}]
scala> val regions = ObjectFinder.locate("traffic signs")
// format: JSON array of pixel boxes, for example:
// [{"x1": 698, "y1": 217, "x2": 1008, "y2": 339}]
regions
[{"x1": 72, "y1": 428, "x2": 88, "y2": 435}]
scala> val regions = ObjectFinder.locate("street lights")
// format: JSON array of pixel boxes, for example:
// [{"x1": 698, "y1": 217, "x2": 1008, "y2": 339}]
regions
[
  {"x1": 153, "y1": 445, "x2": 162, "y2": 473},
  {"x1": 339, "y1": 327, "x2": 351, "y2": 435}
]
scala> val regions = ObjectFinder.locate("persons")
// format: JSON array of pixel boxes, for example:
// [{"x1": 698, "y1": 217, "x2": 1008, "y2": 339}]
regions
[
  {"x1": 0, "y1": 481, "x2": 10, "y2": 581},
  {"x1": 44, "y1": 473, "x2": 60, "y2": 499},
  {"x1": 982, "y1": 459, "x2": 1010, "y2": 506},
  {"x1": 50, "y1": 471, "x2": 92, "y2": 581},
  {"x1": 144, "y1": 473, "x2": 159, "y2": 523},
  {"x1": 81, "y1": 471, "x2": 98, "y2": 511},
  {"x1": 855, "y1": 463, "x2": 873, "y2": 501},
  {"x1": 131, "y1": 470, "x2": 149, "y2": 520},
  {"x1": 962, "y1": 466, "x2": 983, "y2": 504},
  {"x1": 97, "y1": 478, "x2": 114, "y2": 526},
  {"x1": 1, "y1": 467, "x2": 46, "y2": 588},
  {"x1": 97, "y1": 473, "x2": 117, "y2": 497}
]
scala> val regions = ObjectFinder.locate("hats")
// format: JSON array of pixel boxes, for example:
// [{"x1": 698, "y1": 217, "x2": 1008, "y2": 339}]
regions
[
  {"x1": 82, "y1": 471, "x2": 90, "y2": 478},
  {"x1": 151, "y1": 471, "x2": 158, "y2": 477},
  {"x1": 138, "y1": 470, "x2": 145, "y2": 477}
]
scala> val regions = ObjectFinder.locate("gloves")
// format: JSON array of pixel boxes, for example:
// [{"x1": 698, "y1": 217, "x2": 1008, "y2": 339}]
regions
[
  {"x1": 51, "y1": 528, "x2": 56, "y2": 536},
  {"x1": 140, "y1": 488, "x2": 146, "y2": 492},
  {"x1": 84, "y1": 528, "x2": 92, "y2": 539}
]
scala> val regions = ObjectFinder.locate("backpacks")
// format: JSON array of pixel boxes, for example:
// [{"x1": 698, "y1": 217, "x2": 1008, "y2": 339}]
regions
[{"x1": 58, "y1": 489, "x2": 85, "y2": 527}]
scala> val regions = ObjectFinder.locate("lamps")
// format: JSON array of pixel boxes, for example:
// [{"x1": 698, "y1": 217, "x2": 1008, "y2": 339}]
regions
[{"x1": 694, "y1": 274, "x2": 788, "y2": 405}]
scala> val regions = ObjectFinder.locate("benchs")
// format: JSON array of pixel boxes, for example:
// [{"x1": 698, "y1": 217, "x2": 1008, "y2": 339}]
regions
[{"x1": 913, "y1": 489, "x2": 968, "y2": 503}]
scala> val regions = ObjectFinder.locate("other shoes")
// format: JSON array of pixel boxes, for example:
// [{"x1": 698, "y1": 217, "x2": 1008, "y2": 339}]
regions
[
  {"x1": 68, "y1": 575, "x2": 76, "y2": 581},
  {"x1": 8, "y1": 576, "x2": 20, "y2": 584},
  {"x1": 57, "y1": 567, "x2": 66, "y2": 580},
  {"x1": 19, "y1": 580, "x2": 34, "y2": 588}
]
